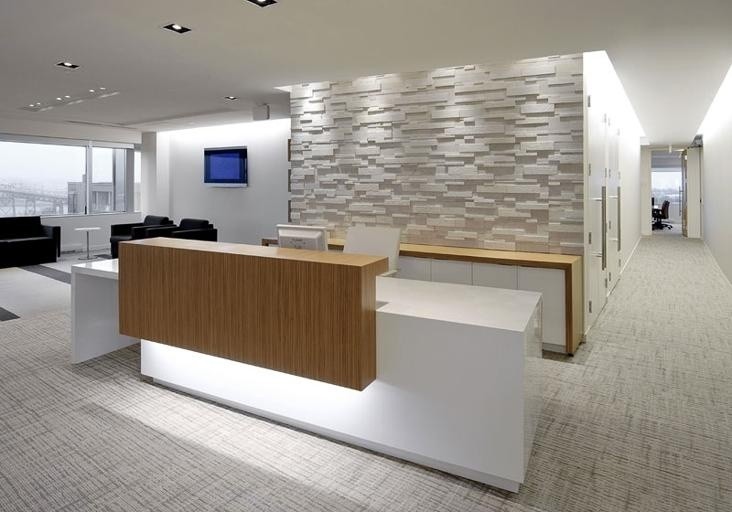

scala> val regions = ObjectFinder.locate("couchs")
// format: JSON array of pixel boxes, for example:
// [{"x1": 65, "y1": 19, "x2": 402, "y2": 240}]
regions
[{"x1": 1, "y1": 215, "x2": 61, "y2": 269}]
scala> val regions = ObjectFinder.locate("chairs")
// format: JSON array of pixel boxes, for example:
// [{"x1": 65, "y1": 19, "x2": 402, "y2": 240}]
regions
[
  {"x1": 108, "y1": 213, "x2": 176, "y2": 258},
  {"x1": 652, "y1": 200, "x2": 673, "y2": 230},
  {"x1": 146, "y1": 218, "x2": 217, "y2": 242},
  {"x1": 341, "y1": 225, "x2": 400, "y2": 277}
]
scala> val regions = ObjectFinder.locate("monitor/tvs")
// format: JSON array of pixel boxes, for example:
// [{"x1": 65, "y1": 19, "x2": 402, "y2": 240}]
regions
[
  {"x1": 202, "y1": 146, "x2": 250, "y2": 188},
  {"x1": 275, "y1": 223, "x2": 328, "y2": 252}
]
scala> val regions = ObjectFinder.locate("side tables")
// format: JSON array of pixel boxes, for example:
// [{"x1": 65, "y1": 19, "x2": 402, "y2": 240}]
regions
[{"x1": 74, "y1": 227, "x2": 102, "y2": 261}]
